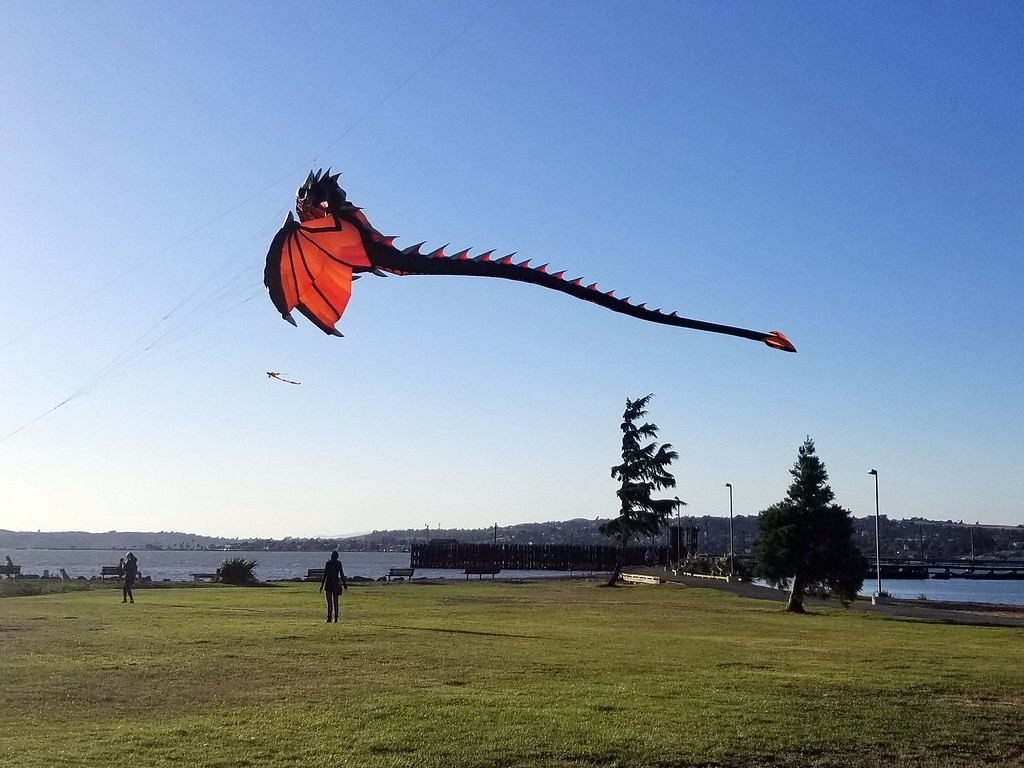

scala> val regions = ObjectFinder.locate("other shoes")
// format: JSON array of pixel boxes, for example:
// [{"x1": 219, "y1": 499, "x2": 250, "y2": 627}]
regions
[
  {"x1": 121, "y1": 600, "x2": 127, "y2": 603},
  {"x1": 333, "y1": 619, "x2": 338, "y2": 622},
  {"x1": 325, "y1": 619, "x2": 331, "y2": 622}
]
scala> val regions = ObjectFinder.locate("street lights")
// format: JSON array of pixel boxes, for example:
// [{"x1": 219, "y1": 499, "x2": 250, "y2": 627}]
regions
[
  {"x1": 867, "y1": 468, "x2": 890, "y2": 606},
  {"x1": 725, "y1": 483, "x2": 738, "y2": 583},
  {"x1": 673, "y1": 496, "x2": 684, "y2": 575}
]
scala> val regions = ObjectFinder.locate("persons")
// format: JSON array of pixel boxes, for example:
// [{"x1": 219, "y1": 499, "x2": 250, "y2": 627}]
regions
[
  {"x1": 5, "y1": 556, "x2": 14, "y2": 579},
  {"x1": 318, "y1": 551, "x2": 348, "y2": 622},
  {"x1": 120, "y1": 551, "x2": 138, "y2": 603}
]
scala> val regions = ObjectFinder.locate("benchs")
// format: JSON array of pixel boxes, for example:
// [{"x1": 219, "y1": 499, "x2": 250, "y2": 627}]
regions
[
  {"x1": 304, "y1": 569, "x2": 325, "y2": 579},
  {"x1": 189, "y1": 574, "x2": 220, "y2": 582},
  {"x1": 385, "y1": 568, "x2": 415, "y2": 581},
  {"x1": 0, "y1": 566, "x2": 21, "y2": 580},
  {"x1": 461, "y1": 566, "x2": 500, "y2": 579},
  {"x1": 100, "y1": 566, "x2": 137, "y2": 582}
]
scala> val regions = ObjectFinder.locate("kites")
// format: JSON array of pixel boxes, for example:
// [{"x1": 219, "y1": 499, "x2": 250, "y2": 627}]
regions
[
  {"x1": 263, "y1": 167, "x2": 797, "y2": 353},
  {"x1": 266, "y1": 372, "x2": 301, "y2": 385}
]
